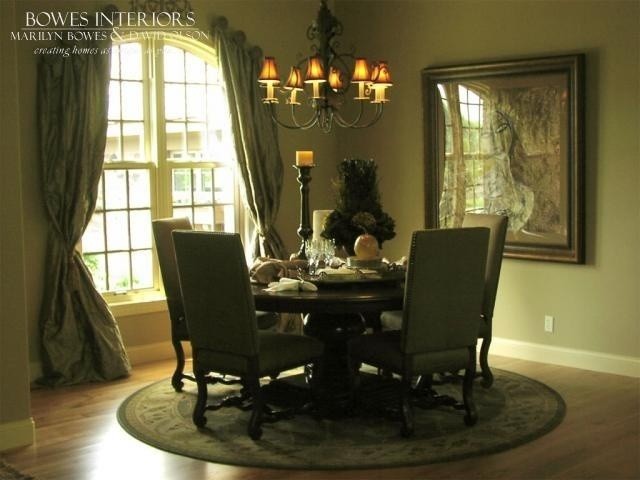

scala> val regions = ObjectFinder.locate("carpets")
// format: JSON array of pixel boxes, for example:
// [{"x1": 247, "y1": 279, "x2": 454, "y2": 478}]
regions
[{"x1": 118, "y1": 360, "x2": 568, "y2": 471}]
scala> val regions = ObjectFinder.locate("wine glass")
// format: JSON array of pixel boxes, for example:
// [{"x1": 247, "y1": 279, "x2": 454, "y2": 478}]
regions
[{"x1": 305, "y1": 238, "x2": 336, "y2": 276}]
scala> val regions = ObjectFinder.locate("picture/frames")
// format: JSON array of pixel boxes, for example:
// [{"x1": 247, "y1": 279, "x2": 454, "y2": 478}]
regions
[{"x1": 420, "y1": 50, "x2": 586, "y2": 268}]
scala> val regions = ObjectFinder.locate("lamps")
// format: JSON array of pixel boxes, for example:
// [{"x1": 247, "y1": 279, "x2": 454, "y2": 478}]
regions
[{"x1": 250, "y1": 0, "x2": 394, "y2": 135}]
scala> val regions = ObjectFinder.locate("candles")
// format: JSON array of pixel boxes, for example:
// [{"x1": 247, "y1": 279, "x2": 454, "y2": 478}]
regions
[{"x1": 295, "y1": 150, "x2": 315, "y2": 168}]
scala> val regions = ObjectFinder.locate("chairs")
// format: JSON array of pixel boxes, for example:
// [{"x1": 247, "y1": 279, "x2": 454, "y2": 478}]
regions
[{"x1": 146, "y1": 210, "x2": 508, "y2": 441}]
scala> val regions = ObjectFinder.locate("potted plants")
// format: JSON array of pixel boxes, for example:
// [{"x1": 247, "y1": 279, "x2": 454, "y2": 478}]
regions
[{"x1": 318, "y1": 155, "x2": 397, "y2": 256}]
